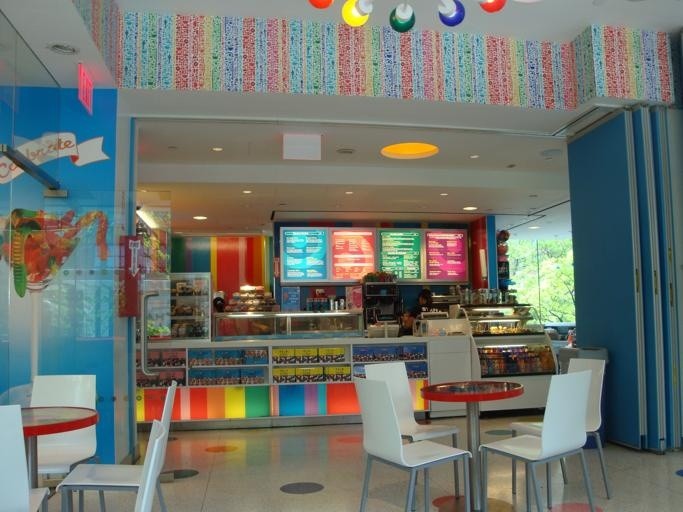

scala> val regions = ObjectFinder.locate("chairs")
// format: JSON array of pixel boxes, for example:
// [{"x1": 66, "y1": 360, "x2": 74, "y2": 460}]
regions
[
  {"x1": 354, "y1": 356, "x2": 611, "y2": 511},
  {"x1": 0, "y1": 375, "x2": 178, "y2": 511}
]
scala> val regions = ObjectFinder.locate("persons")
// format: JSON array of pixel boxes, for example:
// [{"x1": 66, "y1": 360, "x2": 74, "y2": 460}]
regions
[{"x1": 400, "y1": 289, "x2": 432, "y2": 335}]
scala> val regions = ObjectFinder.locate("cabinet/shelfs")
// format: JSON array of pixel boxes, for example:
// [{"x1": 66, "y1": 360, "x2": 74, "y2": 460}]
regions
[
  {"x1": 171, "y1": 273, "x2": 212, "y2": 345},
  {"x1": 460, "y1": 303, "x2": 562, "y2": 419}
]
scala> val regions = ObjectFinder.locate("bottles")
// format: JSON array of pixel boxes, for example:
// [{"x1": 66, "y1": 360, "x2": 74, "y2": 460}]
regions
[
  {"x1": 306, "y1": 297, "x2": 345, "y2": 311},
  {"x1": 450, "y1": 285, "x2": 518, "y2": 306},
  {"x1": 567, "y1": 327, "x2": 576, "y2": 348}
]
